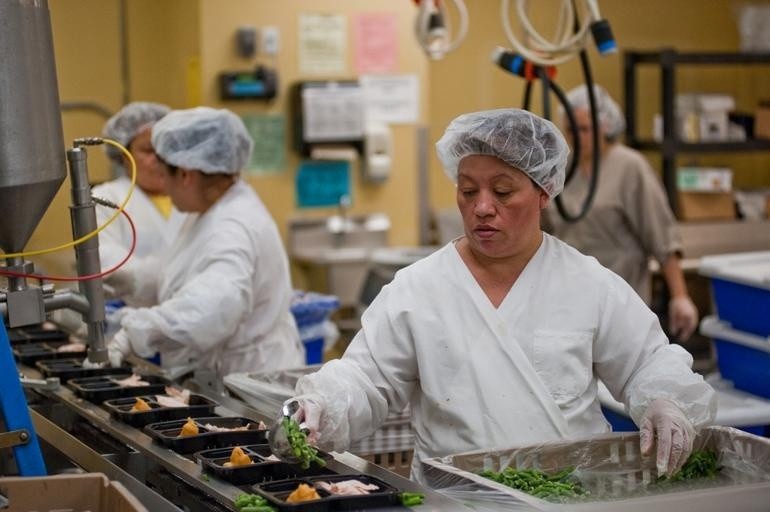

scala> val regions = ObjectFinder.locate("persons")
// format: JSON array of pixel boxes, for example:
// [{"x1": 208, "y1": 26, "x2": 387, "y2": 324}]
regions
[
  {"x1": 78, "y1": 105, "x2": 310, "y2": 399},
  {"x1": 276, "y1": 107, "x2": 720, "y2": 494},
  {"x1": 83, "y1": 102, "x2": 219, "y2": 307},
  {"x1": 540, "y1": 84, "x2": 702, "y2": 344}
]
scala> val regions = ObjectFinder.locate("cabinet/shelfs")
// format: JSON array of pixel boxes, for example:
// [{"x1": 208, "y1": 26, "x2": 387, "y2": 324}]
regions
[{"x1": 614, "y1": 42, "x2": 770, "y2": 227}]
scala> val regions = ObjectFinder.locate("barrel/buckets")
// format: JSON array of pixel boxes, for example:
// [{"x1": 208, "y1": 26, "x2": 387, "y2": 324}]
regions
[{"x1": 290, "y1": 289, "x2": 340, "y2": 365}]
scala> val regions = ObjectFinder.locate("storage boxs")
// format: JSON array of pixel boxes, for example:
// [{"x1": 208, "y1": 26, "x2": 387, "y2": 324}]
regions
[
  {"x1": 96, "y1": 281, "x2": 338, "y2": 372},
  {"x1": 696, "y1": 306, "x2": 768, "y2": 402},
  {"x1": 670, "y1": 94, "x2": 735, "y2": 143},
  {"x1": 677, "y1": 187, "x2": 738, "y2": 222},
  {"x1": 693, "y1": 248, "x2": 768, "y2": 338},
  {"x1": 1, "y1": 467, "x2": 151, "y2": 511}
]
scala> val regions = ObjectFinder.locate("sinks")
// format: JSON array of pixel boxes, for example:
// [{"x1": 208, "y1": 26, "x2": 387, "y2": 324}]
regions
[{"x1": 295, "y1": 247, "x2": 391, "y2": 305}]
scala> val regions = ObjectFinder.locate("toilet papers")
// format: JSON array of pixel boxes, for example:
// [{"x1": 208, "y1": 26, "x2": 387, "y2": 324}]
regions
[{"x1": 367, "y1": 156, "x2": 391, "y2": 179}]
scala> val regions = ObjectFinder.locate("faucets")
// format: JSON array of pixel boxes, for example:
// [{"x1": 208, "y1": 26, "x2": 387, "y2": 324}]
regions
[{"x1": 331, "y1": 194, "x2": 358, "y2": 244}]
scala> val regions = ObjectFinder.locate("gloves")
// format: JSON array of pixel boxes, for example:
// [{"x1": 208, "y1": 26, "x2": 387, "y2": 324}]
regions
[{"x1": 82, "y1": 331, "x2": 128, "y2": 369}]
